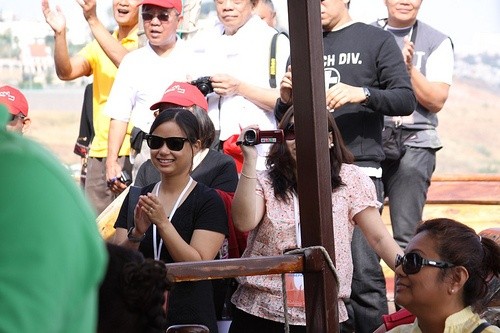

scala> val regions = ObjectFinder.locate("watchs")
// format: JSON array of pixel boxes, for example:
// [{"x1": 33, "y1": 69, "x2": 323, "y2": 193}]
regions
[{"x1": 364, "y1": 88, "x2": 370, "y2": 100}]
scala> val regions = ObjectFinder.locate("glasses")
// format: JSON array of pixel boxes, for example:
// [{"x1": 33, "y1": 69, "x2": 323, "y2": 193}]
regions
[
  {"x1": 153, "y1": 106, "x2": 185, "y2": 118},
  {"x1": 394, "y1": 252, "x2": 455, "y2": 275},
  {"x1": 145, "y1": 135, "x2": 191, "y2": 151},
  {"x1": 283, "y1": 123, "x2": 332, "y2": 140},
  {"x1": 142, "y1": 12, "x2": 179, "y2": 21},
  {"x1": 8, "y1": 114, "x2": 24, "y2": 121}
]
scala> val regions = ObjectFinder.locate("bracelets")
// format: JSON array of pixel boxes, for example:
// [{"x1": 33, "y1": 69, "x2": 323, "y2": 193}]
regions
[
  {"x1": 127, "y1": 226, "x2": 146, "y2": 243},
  {"x1": 240, "y1": 170, "x2": 257, "y2": 179}
]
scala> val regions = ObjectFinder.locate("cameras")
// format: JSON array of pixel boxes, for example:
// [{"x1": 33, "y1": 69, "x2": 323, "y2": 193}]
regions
[
  {"x1": 243, "y1": 128, "x2": 285, "y2": 145},
  {"x1": 190, "y1": 76, "x2": 214, "y2": 96}
]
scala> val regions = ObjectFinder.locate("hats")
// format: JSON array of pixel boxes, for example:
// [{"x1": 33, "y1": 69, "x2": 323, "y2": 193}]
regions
[
  {"x1": 136, "y1": 0, "x2": 182, "y2": 14},
  {"x1": 0, "y1": 85, "x2": 28, "y2": 117},
  {"x1": 149, "y1": 82, "x2": 209, "y2": 112}
]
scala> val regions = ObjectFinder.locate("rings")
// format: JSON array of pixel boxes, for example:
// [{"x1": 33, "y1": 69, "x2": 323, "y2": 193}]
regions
[{"x1": 150, "y1": 208, "x2": 153, "y2": 210}]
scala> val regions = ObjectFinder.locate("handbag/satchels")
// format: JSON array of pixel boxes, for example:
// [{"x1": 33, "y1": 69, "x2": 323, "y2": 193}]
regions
[
  {"x1": 130, "y1": 127, "x2": 145, "y2": 154},
  {"x1": 381, "y1": 121, "x2": 406, "y2": 161}
]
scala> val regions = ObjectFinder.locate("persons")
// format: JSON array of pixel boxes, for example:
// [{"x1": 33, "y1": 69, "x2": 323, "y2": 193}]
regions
[
  {"x1": 373, "y1": 218, "x2": 500, "y2": 333},
  {"x1": 0, "y1": 85, "x2": 32, "y2": 137},
  {"x1": 369, "y1": 0, "x2": 455, "y2": 315},
  {"x1": 276, "y1": 0, "x2": 414, "y2": 333},
  {"x1": 108, "y1": 107, "x2": 230, "y2": 333},
  {"x1": 230, "y1": 104, "x2": 406, "y2": 332},
  {"x1": 0, "y1": 104, "x2": 108, "y2": 333},
  {"x1": 42, "y1": 0, "x2": 291, "y2": 215}
]
